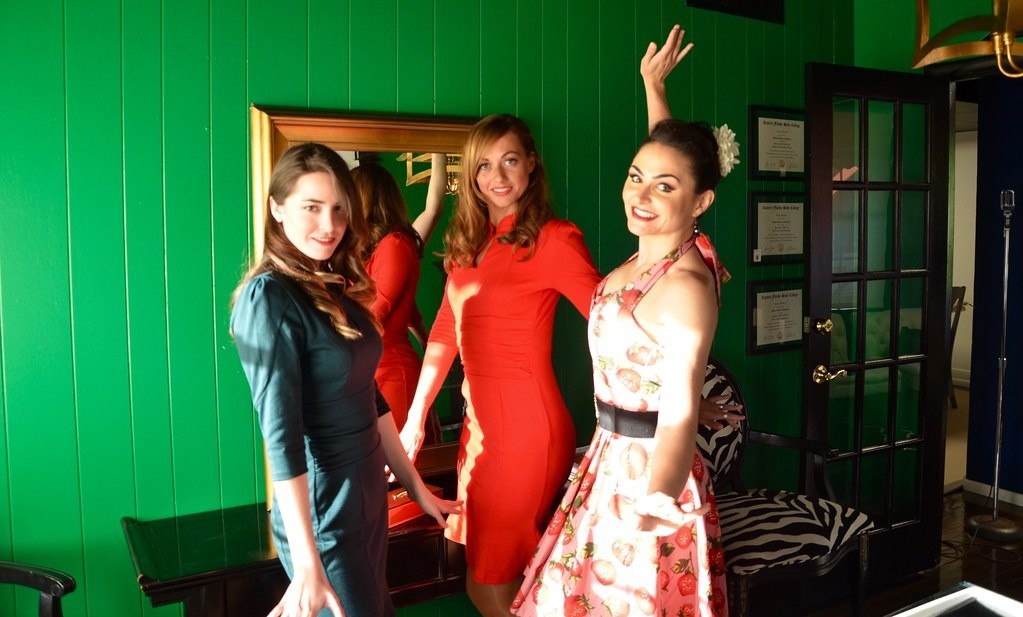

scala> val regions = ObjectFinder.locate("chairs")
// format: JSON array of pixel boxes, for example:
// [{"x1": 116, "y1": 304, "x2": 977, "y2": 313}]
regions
[
  {"x1": 0, "y1": 560, "x2": 78, "y2": 617},
  {"x1": 945, "y1": 287, "x2": 967, "y2": 408},
  {"x1": 696, "y1": 356, "x2": 873, "y2": 616}
]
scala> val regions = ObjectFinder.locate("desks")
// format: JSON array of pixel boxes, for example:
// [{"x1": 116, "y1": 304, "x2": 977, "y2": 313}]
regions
[{"x1": 121, "y1": 489, "x2": 466, "y2": 617}]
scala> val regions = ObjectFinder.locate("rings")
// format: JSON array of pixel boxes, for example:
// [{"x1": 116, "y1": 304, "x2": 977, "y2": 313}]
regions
[
  {"x1": 719, "y1": 404, "x2": 723, "y2": 409},
  {"x1": 723, "y1": 410, "x2": 727, "y2": 418}
]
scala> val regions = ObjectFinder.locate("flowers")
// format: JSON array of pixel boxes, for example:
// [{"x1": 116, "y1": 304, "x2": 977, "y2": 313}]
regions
[{"x1": 712, "y1": 123, "x2": 742, "y2": 178}]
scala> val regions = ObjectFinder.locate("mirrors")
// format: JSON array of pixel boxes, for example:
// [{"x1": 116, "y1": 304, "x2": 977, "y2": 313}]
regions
[{"x1": 249, "y1": 104, "x2": 543, "y2": 513}]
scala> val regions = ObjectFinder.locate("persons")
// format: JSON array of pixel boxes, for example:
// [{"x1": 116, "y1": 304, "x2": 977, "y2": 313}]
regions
[
  {"x1": 231, "y1": 143, "x2": 466, "y2": 617},
  {"x1": 349, "y1": 154, "x2": 448, "y2": 445},
  {"x1": 512, "y1": 24, "x2": 740, "y2": 616},
  {"x1": 385, "y1": 114, "x2": 745, "y2": 616}
]
